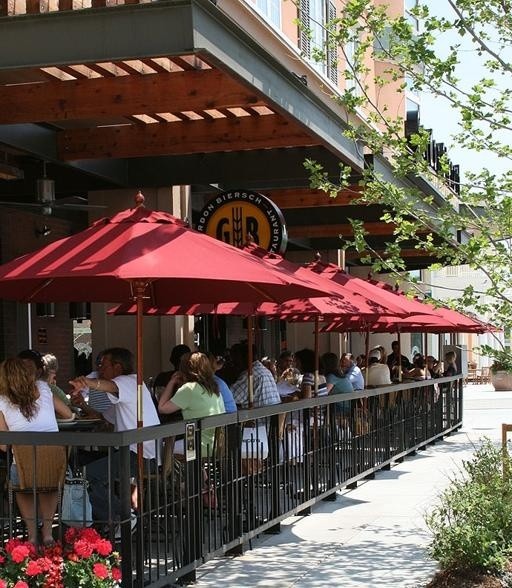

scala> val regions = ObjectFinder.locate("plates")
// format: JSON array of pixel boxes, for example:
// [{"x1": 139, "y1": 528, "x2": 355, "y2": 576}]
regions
[{"x1": 57, "y1": 418, "x2": 103, "y2": 427}]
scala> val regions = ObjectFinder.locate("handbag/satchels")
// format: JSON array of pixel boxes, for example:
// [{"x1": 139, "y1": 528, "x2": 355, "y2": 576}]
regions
[{"x1": 60, "y1": 468, "x2": 94, "y2": 527}]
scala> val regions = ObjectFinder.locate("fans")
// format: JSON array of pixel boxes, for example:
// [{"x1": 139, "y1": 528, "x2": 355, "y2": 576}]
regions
[{"x1": 0, "y1": 159, "x2": 108, "y2": 215}]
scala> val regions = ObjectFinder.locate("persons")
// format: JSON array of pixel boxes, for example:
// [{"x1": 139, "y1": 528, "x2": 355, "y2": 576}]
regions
[{"x1": 1, "y1": 336, "x2": 460, "y2": 551}]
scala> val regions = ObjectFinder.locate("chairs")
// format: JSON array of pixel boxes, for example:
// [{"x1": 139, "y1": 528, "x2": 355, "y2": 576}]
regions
[{"x1": 14, "y1": 354, "x2": 461, "y2": 549}]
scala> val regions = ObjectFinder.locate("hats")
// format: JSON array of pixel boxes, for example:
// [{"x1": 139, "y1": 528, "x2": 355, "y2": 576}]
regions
[{"x1": 370, "y1": 350, "x2": 381, "y2": 360}]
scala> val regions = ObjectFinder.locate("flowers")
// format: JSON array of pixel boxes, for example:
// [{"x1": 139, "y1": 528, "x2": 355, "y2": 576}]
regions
[{"x1": 0, "y1": 530, "x2": 122, "y2": 588}]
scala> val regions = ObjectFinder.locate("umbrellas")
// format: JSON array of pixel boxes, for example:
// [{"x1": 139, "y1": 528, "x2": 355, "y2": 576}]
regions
[
  {"x1": 106, "y1": 238, "x2": 408, "y2": 411},
  {"x1": 0, "y1": 189, "x2": 345, "y2": 515}
]
